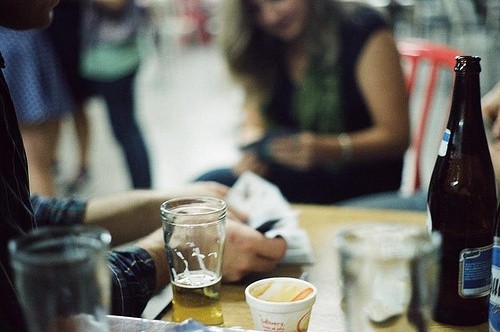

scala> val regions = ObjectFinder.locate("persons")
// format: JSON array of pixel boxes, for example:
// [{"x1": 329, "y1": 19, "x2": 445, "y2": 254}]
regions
[
  {"x1": 0, "y1": 0, "x2": 286, "y2": 332},
  {"x1": 57, "y1": 1, "x2": 162, "y2": 190},
  {"x1": 194, "y1": 0, "x2": 411, "y2": 207},
  {"x1": 0, "y1": 0, "x2": 74, "y2": 201}
]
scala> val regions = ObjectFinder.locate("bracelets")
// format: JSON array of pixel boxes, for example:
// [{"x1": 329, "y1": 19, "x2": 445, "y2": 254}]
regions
[{"x1": 336, "y1": 133, "x2": 353, "y2": 163}]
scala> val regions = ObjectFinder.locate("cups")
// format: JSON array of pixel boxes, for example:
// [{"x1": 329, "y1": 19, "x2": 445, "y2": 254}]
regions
[
  {"x1": 339, "y1": 224, "x2": 444, "y2": 332},
  {"x1": 160, "y1": 196, "x2": 228, "y2": 327},
  {"x1": 9, "y1": 226, "x2": 111, "y2": 332},
  {"x1": 245, "y1": 277, "x2": 317, "y2": 331}
]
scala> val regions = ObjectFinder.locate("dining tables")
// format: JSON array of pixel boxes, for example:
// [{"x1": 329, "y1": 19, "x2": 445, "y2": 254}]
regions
[{"x1": 154, "y1": 204, "x2": 489, "y2": 332}]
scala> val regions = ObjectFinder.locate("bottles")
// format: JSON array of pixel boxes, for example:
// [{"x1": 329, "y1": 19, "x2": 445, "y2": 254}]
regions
[
  {"x1": 428, "y1": 56, "x2": 497, "y2": 328},
  {"x1": 488, "y1": 191, "x2": 500, "y2": 332}
]
